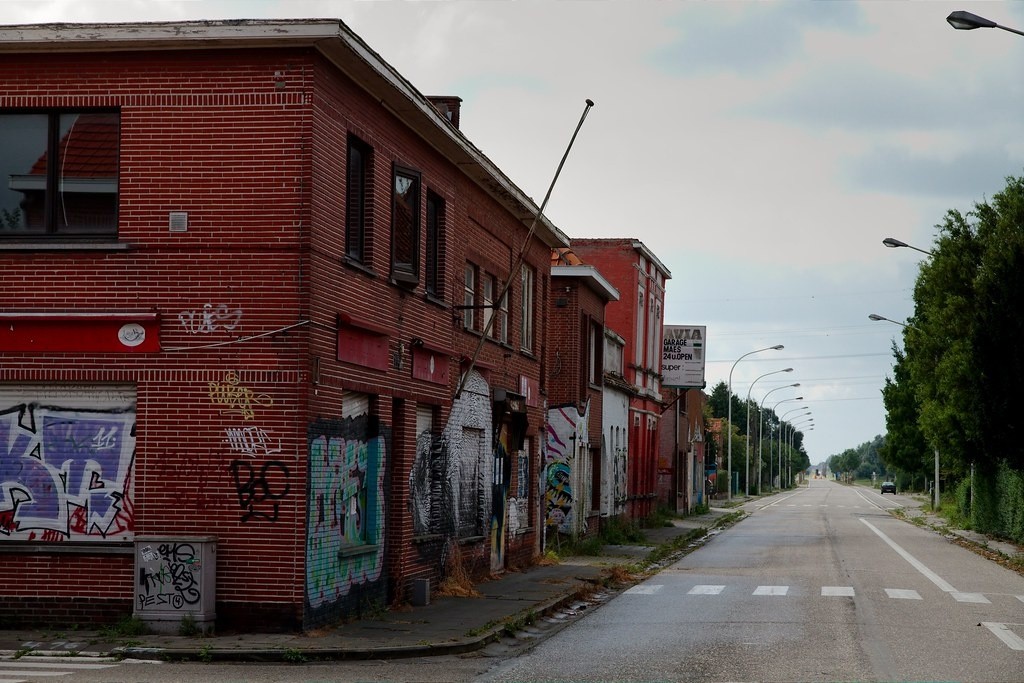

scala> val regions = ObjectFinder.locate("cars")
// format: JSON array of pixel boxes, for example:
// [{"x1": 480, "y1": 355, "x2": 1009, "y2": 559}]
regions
[{"x1": 880, "y1": 482, "x2": 896, "y2": 495}]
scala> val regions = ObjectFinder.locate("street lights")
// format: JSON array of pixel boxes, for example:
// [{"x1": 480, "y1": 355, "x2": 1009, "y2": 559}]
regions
[
  {"x1": 726, "y1": 344, "x2": 785, "y2": 503},
  {"x1": 783, "y1": 412, "x2": 812, "y2": 487},
  {"x1": 868, "y1": 314, "x2": 941, "y2": 512},
  {"x1": 777, "y1": 406, "x2": 808, "y2": 491},
  {"x1": 769, "y1": 396, "x2": 804, "y2": 492},
  {"x1": 789, "y1": 418, "x2": 815, "y2": 486},
  {"x1": 745, "y1": 367, "x2": 793, "y2": 496},
  {"x1": 882, "y1": 237, "x2": 980, "y2": 526},
  {"x1": 758, "y1": 382, "x2": 800, "y2": 494}
]
